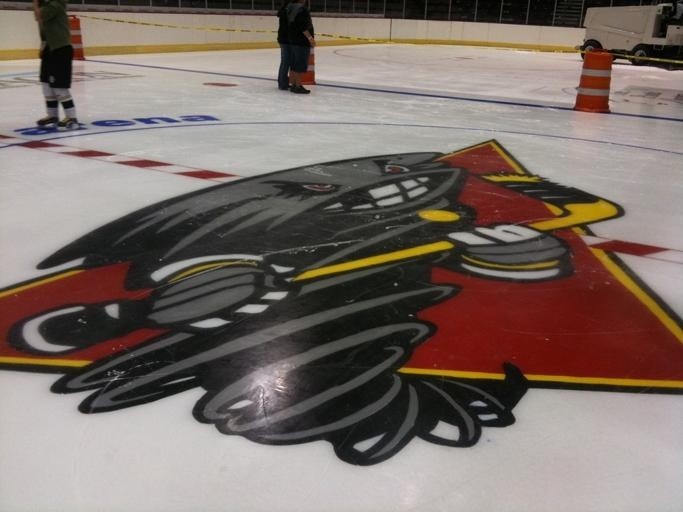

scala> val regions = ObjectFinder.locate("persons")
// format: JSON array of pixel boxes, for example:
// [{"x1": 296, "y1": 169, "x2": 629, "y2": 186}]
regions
[
  {"x1": 286, "y1": 0, "x2": 315, "y2": 93},
  {"x1": 277, "y1": 0, "x2": 291, "y2": 90},
  {"x1": 33, "y1": 0, "x2": 77, "y2": 127}
]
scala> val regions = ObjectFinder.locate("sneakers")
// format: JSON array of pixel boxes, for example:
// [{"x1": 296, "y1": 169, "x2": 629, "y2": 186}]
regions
[
  {"x1": 38, "y1": 116, "x2": 56, "y2": 125},
  {"x1": 279, "y1": 84, "x2": 310, "y2": 93},
  {"x1": 58, "y1": 117, "x2": 77, "y2": 126}
]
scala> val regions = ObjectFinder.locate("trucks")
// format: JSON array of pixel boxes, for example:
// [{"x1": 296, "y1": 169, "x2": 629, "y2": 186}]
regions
[{"x1": 575, "y1": 0, "x2": 683, "y2": 70}]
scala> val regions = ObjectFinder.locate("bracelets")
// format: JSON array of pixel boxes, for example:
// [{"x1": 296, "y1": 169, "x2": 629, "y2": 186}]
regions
[{"x1": 308, "y1": 36, "x2": 312, "y2": 41}]
